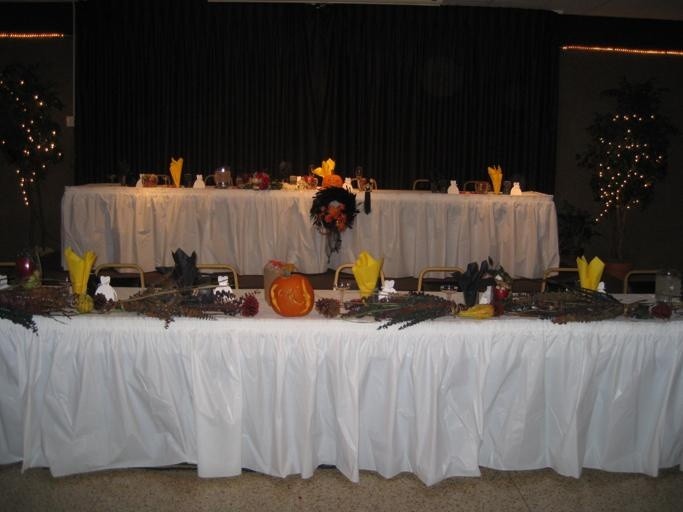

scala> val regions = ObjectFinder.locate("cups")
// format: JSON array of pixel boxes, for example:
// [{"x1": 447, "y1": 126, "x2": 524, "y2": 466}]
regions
[
  {"x1": 214, "y1": 166, "x2": 232, "y2": 189},
  {"x1": 183, "y1": 172, "x2": 194, "y2": 188},
  {"x1": 502, "y1": 179, "x2": 512, "y2": 194}
]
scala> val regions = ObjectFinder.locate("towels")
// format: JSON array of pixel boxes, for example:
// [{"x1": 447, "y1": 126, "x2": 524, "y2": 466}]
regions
[
  {"x1": 349, "y1": 251, "x2": 383, "y2": 300},
  {"x1": 311, "y1": 155, "x2": 336, "y2": 189},
  {"x1": 486, "y1": 162, "x2": 504, "y2": 196},
  {"x1": 167, "y1": 156, "x2": 185, "y2": 189},
  {"x1": 574, "y1": 255, "x2": 605, "y2": 292},
  {"x1": 63, "y1": 246, "x2": 96, "y2": 306}
]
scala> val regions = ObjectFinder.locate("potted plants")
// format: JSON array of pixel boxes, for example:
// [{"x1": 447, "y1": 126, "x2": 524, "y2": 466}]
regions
[
  {"x1": 571, "y1": 70, "x2": 681, "y2": 283},
  {"x1": 0, "y1": 59, "x2": 67, "y2": 271}
]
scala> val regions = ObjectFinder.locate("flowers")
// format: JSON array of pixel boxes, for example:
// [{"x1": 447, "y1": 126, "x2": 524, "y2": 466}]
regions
[
  {"x1": 308, "y1": 186, "x2": 364, "y2": 263},
  {"x1": 238, "y1": 167, "x2": 283, "y2": 192},
  {"x1": 0, "y1": 268, "x2": 261, "y2": 336}
]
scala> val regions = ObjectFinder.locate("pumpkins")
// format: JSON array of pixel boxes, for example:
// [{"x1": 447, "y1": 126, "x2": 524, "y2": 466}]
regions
[
  {"x1": 269, "y1": 268, "x2": 314, "y2": 317},
  {"x1": 321, "y1": 174, "x2": 342, "y2": 188}
]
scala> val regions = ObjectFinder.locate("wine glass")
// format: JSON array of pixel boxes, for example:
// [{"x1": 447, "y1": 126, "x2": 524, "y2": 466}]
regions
[
  {"x1": 440, "y1": 285, "x2": 459, "y2": 301},
  {"x1": 333, "y1": 279, "x2": 351, "y2": 308}
]
